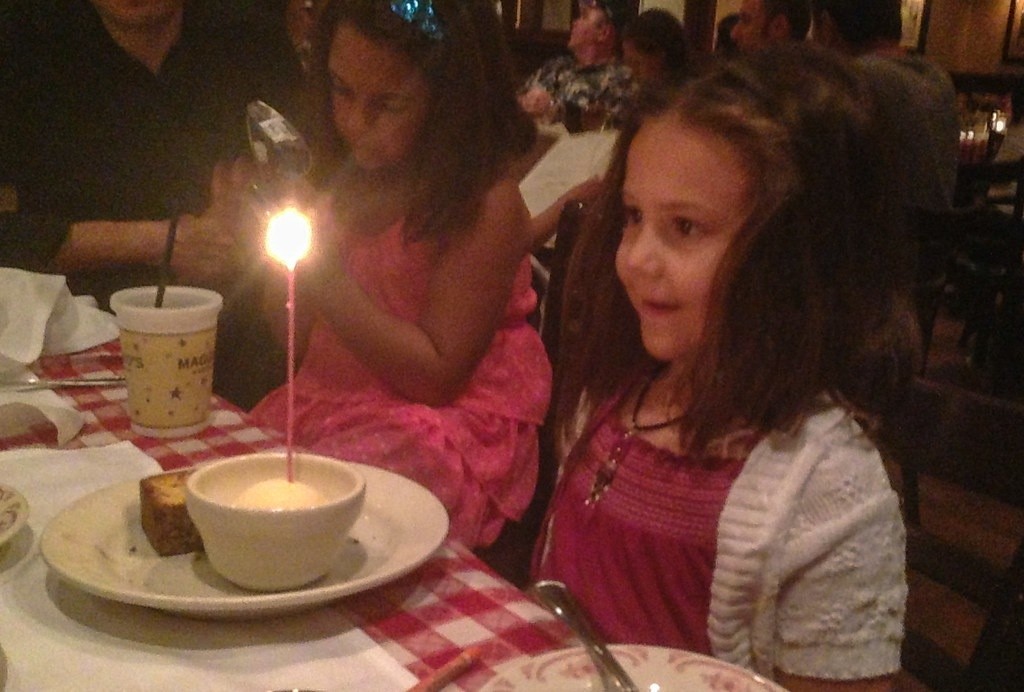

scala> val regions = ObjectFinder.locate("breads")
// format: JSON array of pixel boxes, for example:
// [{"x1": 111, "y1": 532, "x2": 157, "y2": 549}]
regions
[{"x1": 137, "y1": 469, "x2": 207, "y2": 557}]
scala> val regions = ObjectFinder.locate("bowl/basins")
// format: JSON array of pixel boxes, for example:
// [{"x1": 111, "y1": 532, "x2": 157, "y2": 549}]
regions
[{"x1": 184, "y1": 452, "x2": 366, "y2": 590}]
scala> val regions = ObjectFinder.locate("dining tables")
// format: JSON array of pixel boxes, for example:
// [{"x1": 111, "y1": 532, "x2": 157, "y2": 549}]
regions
[{"x1": 0, "y1": 340, "x2": 581, "y2": 692}]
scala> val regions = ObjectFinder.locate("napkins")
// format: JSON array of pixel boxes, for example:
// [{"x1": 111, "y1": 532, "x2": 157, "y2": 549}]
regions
[
  {"x1": 0, "y1": 368, "x2": 86, "y2": 448},
  {"x1": 0, "y1": 267, "x2": 120, "y2": 374}
]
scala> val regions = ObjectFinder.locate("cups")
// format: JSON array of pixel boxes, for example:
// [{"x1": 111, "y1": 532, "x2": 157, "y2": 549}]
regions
[{"x1": 109, "y1": 286, "x2": 223, "y2": 438}]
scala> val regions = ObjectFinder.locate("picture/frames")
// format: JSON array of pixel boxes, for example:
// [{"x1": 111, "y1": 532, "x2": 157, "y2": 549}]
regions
[
  {"x1": 999, "y1": 0, "x2": 1024, "y2": 66},
  {"x1": 638, "y1": 0, "x2": 686, "y2": 29},
  {"x1": 899, "y1": 0, "x2": 932, "y2": 55}
]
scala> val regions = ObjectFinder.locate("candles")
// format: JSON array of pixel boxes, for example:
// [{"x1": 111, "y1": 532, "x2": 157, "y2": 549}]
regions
[{"x1": 266, "y1": 205, "x2": 313, "y2": 483}]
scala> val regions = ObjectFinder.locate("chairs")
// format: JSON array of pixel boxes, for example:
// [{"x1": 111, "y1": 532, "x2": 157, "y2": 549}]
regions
[
  {"x1": 875, "y1": 378, "x2": 1024, "y2": 692},
  {"x1": 897, "y1": 69, "x2": 1023, "y2": 528}
]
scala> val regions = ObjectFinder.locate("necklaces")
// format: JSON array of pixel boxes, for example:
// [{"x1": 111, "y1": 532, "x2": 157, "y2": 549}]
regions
[{"x1": 588, "y1": 358, "x2": 694, "y2": 505}]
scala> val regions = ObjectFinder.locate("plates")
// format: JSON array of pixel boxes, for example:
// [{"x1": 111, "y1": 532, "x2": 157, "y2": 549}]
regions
[
  {"x1": 0, "y1": 402, "x2": 86, "y2": 454},
  {"x1": 39, "y1": 461, "x2": 450, "y2": 617},
  {"x1": 469, "y1": 642, "x2": 794, "y2": 692}
]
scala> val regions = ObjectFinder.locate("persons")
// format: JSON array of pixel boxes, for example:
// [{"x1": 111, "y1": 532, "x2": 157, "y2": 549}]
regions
[
  {"x1": 513, "y1": 0, "x2": 698, "y2": 134},
  {"x1": 527, "y1": 63, "x2": 910, "y2": 692},
  {"x1": 712, "y1": 0, "x2": 963, "y2": 293},
  {"x1": 0, "y1": 1, "x2": 317, "y2": 410},
  {"x1": 251, "y1": 0, "x2": 548, "y2": 557}
]
